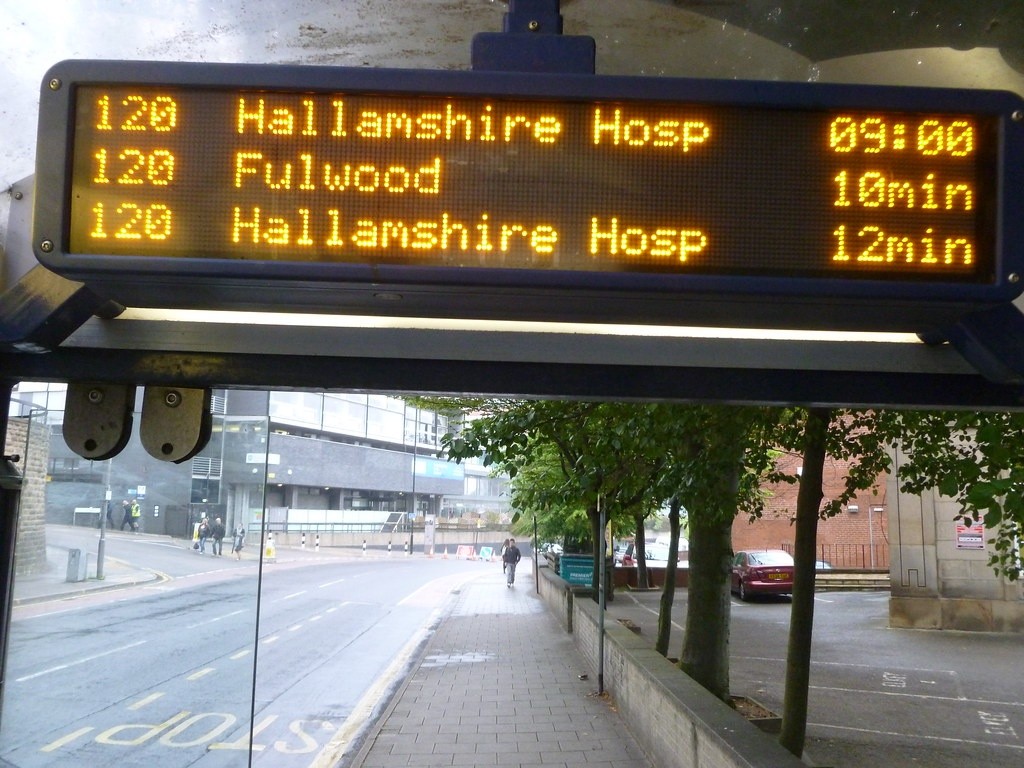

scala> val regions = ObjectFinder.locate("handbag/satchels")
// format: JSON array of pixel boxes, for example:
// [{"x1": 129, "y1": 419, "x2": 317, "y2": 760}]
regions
[{"x1": 193, "y1": 540, "x2": 200, "y2": 549}]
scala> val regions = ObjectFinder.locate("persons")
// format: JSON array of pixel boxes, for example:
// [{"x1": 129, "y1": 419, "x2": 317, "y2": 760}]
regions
[
  {"x1": 121, "y1": 500, "x2": 140, "y2": 532},
  {"x1": 233, "y1": 523, "x2": 245, "y2": 561},
  {"x1": 97, "y1": 500, "x2": 115, "y2": 529},
  {"x1": 198, "y1": 518, "x2": 225, "y2": 557},
  {"x1": 501, "y1": 539, "x2": 521, "y2": 587}
]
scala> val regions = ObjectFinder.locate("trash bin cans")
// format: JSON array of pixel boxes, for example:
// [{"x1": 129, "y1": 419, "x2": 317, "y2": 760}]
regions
[
  {"x1": 66, "y1": 548, "x2": 87, "y2": 582},
  {"x1": 73, "y1": 507, "x2": 101, "y2": 529}
]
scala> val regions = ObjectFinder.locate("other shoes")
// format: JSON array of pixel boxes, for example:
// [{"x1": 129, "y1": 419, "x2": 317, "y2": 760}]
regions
[
  {"x1": 507, "y1": 583, "x2": 510, "y2": 588},
  {"x1": 511, "y1": 583, "x2": 514, "y2": 588},
  {"x1": 200, "y1": 552, "x2": 203, "y2": 555}
]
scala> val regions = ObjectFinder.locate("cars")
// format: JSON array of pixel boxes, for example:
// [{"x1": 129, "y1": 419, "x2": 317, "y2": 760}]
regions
[
  {"x1": 731, "y1": 550, "x2": 793, "y2": 602},
  {"x1": 615, "y1": 545, "x2": 628, "y2": 562},
  {"x1": 623, "y1": 543, "x2": 680, "y2": 569},
  {"x1": 537, "y1": 543, "x2": 562, "y2": 556}
]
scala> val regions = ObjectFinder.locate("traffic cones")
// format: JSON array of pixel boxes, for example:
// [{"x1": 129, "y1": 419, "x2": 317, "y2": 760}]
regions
[
  {"x1": 441, "y1": 548, "x2": 448, "y2": 558},
  {"x1": 427, "y1": 548, "x2": 435, "y2": 558}
]
